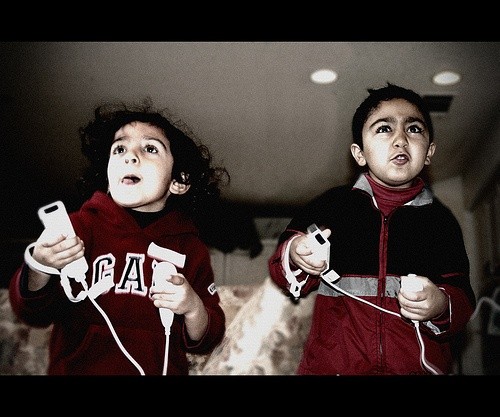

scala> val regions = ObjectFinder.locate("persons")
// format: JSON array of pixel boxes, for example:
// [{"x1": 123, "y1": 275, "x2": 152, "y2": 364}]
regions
[
  {"x1": 265, "y1": 82, "x2": 475, "y2": 375},
  {"x1": 8, "y1": 96, "x2": 230, "y2": 377}
]
